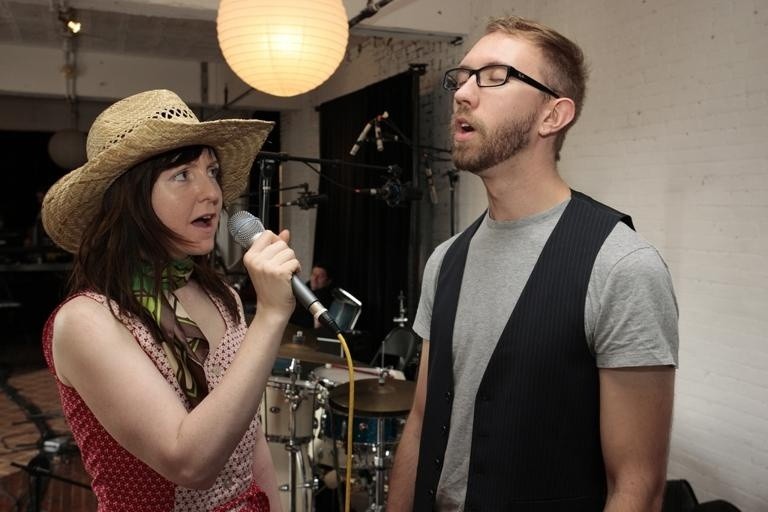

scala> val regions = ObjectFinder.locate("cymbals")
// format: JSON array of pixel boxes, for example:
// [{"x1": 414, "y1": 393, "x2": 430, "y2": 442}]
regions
[
  {"x1": 278, "y1": 343, "x2": 360, "y2": 366},
  {"x1": 328, "y1": 378, "x2": 416, "y2": 412}
]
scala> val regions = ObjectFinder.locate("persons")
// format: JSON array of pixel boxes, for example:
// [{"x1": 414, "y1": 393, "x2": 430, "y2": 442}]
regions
[
  {"x1": 41, "y1": 89, "x2": 303, "y2": 512},
  {"x1": 386, "y1": 14, "x2": 679, "y2": 512},
  {"x1": 305, "y1": 262, "x2": 336, "y2": 308}
]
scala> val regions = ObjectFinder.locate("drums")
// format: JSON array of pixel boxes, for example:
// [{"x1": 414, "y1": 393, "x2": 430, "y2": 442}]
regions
[
  {"x1": 254, "y1": 376, "x2": 319, "y2": 445},
  {"x1": 307, "y1": 364, "x2": 406, "y2": 471},
  {"x1": 267, "y1": 443, "x2": 320, "y2": 512},
  {"x1": 319, "y1": 399, "x2": 407, "y2": 448}
]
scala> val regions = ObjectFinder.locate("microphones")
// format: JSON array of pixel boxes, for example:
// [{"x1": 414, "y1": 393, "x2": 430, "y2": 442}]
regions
[
  {"x1": 425, "y1": 159, "x2": 439, "y2": 205},
  {"x1": 349, "y1": 121, "x2": 373, "y2": 157},
  {"x1": 374, "y1": 120, "x2": 384, "y2": 151},
  {"x1": 227, "y1": 210, "x2": 339, "y2": 332},
  {"x1": 280, "y1": 193, "x2": 327, "y2": 207},
  {"x1": 367, "y1": 184, "x2": 423, "y2": 202}
]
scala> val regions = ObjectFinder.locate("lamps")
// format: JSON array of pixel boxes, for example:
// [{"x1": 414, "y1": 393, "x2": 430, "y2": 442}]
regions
[{"x1": 216, "y1": 0, "x2": 349, "y2": 99}]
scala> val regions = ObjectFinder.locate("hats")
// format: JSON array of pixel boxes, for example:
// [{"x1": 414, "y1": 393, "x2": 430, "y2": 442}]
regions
[{"x1": 41, "y1": 89, "x2": 275, "y2": 254}]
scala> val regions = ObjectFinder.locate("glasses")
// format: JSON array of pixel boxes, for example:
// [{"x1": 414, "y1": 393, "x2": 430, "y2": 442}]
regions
[{"x1": 440, "y1": 65, "x2": 559, "y2": 98}]
